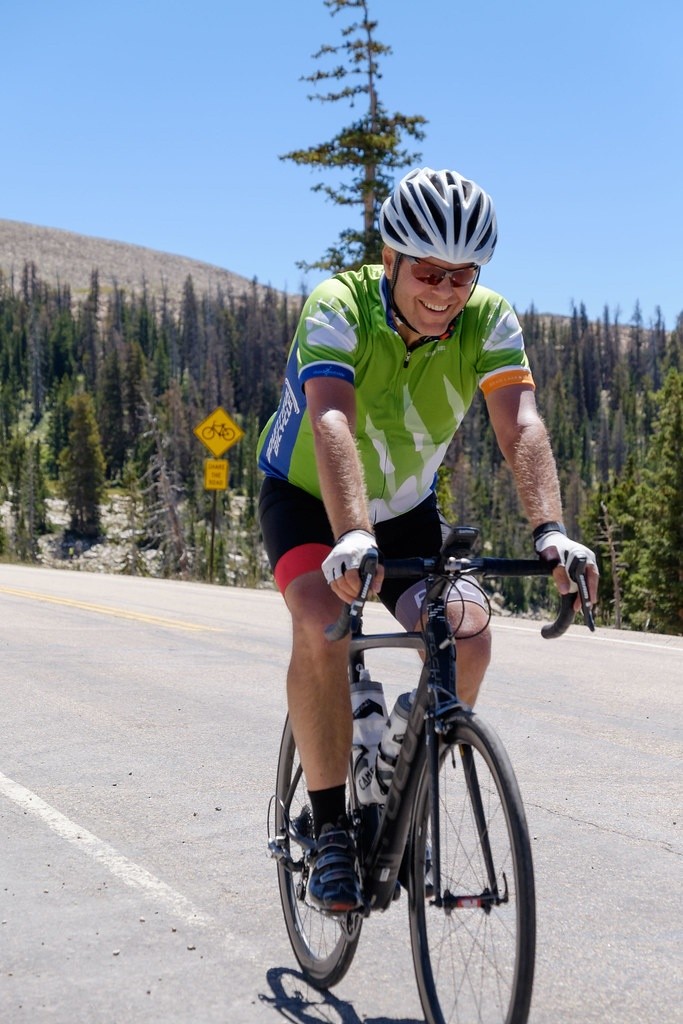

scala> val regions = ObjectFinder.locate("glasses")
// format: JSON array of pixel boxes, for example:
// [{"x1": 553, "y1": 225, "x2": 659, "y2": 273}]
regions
[{"x1": 410, "y1": 257, "x2": 478, "y2": 287}]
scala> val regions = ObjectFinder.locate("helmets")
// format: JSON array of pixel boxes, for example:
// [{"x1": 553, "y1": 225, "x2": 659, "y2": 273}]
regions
[{"x1": 378, "y1": 167, "x2": 497, "y2": 266}]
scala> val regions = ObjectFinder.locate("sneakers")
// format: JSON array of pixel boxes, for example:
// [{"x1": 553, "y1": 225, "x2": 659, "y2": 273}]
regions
[
  {"x1": 399, "y1": 829, "x2": 431, "y2": 876},
  {"x1": 304, "y1": 822, "x2": 362, "y2": 913}
]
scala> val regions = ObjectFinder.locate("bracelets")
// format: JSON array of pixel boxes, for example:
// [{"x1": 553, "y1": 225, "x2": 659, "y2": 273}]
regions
[{"x1": 532, "y1": 521, "x2": 567, "y2": 539}]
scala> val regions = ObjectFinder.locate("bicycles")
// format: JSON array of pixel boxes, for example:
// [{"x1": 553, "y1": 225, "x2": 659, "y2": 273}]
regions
[{"x1": 264, "y1": 528, "x2": 598, "y2": 1024}]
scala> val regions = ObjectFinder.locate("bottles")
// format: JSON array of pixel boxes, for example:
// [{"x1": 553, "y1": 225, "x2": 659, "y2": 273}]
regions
[
  {"x1": 372, "y1": 688, "x2": 417, "y2": 804},
  {"x1": 350, "y1": 668, "x2": 390, "y2": 804}
]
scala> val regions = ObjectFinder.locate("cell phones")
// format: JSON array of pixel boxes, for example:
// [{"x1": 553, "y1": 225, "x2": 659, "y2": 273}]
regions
[{"x1": 439, "y1": 526, "x2": 479, "y2": 556}]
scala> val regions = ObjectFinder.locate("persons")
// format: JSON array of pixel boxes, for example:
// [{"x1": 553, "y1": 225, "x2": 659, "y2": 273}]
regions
[{"x1": 257, "y1": 169, "x2": 598, "y2": 914}]
